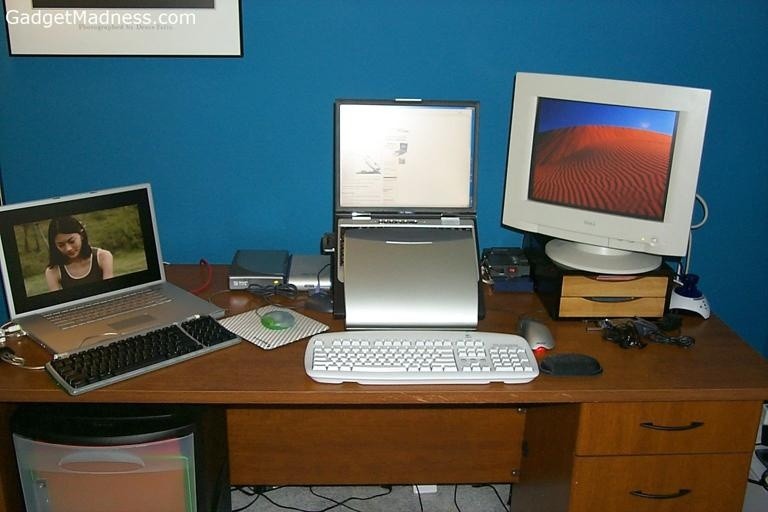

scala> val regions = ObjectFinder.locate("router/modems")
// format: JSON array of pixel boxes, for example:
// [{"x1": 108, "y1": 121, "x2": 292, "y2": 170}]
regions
[{"x1": 227, "y1": 248, "x2": 289, "y2": 291}]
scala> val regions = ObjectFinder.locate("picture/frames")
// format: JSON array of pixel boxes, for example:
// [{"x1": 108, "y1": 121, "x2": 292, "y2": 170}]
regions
[{"x1": 1, "y1": 0, "x2": 244, "y2": 59}]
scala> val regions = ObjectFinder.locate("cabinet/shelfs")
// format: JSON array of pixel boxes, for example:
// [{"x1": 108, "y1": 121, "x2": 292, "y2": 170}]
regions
[{"x1": 11, "y1": 402, "x2": 215, "y2": 512}]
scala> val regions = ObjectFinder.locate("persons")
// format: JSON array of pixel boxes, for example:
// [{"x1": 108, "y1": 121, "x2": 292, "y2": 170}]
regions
[{"x1": 44, "y1": 215, "x2": 114, "y2": 292}]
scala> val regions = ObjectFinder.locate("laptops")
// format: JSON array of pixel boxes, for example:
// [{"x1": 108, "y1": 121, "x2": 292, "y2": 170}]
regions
[{"x1": 0, "y1": 183, "x2": 226, "y2": 356}]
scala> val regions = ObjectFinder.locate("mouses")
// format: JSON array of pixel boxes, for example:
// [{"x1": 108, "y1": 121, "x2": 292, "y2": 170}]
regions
[
  {"x1": 520, "y1": 318, "x2": 556, "y2": 350},
  {"x1": 261, "y1": 311, "x2": 296, "y2": 330}
]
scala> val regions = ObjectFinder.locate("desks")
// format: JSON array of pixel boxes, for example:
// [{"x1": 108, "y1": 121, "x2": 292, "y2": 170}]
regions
[{"x1": 1, "y1": 264, "x2": 767, "y2": 512}]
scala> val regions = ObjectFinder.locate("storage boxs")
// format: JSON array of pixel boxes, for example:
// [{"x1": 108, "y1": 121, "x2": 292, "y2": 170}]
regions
[{"x1": 533, "y1": 252, "x2": 676, "y2": 322}]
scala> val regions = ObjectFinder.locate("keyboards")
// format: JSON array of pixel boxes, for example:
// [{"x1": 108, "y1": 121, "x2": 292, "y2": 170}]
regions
[
  {"x1": 47, "y1": 316, "x2": 242, "y2": 396},
  {"x1": 305, "y1": 331, "x2": 540, "y2": 386}
]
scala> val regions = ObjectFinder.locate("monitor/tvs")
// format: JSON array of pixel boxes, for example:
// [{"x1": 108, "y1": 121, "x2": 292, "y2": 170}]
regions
[
  {"x1": 501, "y1": 71, "x2": 712, "y2": 276},
  {"x1": 334, "y1": 98, "x2": 481, "y2": 213}
]
